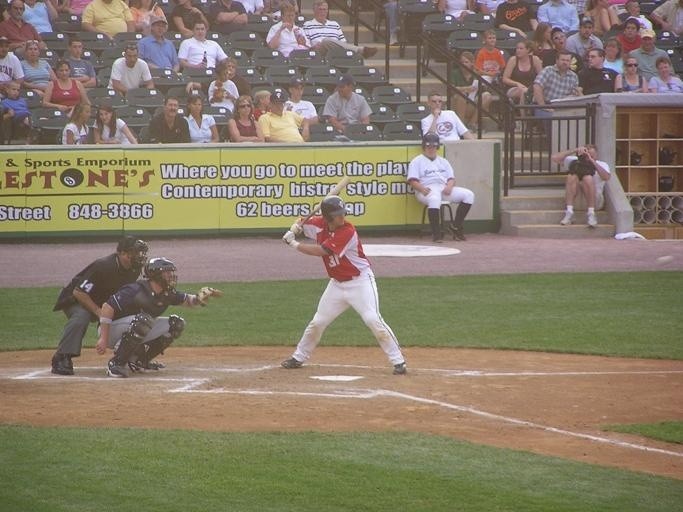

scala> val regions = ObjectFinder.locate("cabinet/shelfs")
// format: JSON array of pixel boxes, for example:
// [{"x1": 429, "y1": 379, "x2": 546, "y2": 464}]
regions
[{"x1": 612, "y1": 104, "x2": 683, "y2": 240}]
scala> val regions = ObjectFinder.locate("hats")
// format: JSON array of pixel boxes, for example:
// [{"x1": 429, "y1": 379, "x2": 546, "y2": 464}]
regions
[
  {"x1": 288, "y1": 78, "x2": 304, "y2": 88},
  {"x1": 270, "y1": 89, "x2": 287, "y2": 103},
  {"x1": 581, "y1": 17, "x2": 593, "y2": 24},
  {"x1": 337, "y1": 75, "x2": 354, "y2": 84},
  {"x1": 152, "y1": 16, "x2": 168, "y2": 27},
  {"x1": 640, "y1": 28, "x2": 657, "y2": 40}
]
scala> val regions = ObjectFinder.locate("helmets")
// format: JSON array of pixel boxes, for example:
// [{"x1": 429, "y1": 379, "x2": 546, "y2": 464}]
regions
[
  {"x1": 321, "y1": 197, "x2": 350, "y2": 217},
  {"x1": 145, "y1": 257, "x2": 177, "y2": 290},
  {"x1": 422, "y1": 133, "x2": 440, "y2": 150},
  {"x1": 117, "y1": 236, "x2": 147, "y2": 269}
]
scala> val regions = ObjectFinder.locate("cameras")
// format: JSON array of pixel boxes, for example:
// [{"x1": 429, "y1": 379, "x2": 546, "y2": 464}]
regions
[{"x1": 578, "y1": 155, "x2": 586, "y2": 161}]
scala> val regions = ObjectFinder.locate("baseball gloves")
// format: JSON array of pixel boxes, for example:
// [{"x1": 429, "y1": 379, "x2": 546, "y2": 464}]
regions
[{"x1": 194, "y1": 287, "x2": 222, "y2": 306}]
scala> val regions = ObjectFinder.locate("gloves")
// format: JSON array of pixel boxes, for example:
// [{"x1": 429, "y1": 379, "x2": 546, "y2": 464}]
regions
[{"x1": 284, "y1": 223, "x2": 302, "y2": 250}]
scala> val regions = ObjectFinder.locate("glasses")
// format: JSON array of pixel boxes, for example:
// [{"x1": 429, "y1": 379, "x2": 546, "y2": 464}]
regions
[
  {"x1": 239, "y1": 104, "x2": 251, "y2": 108},
  {"x1": 627, "y1": 62, "x2": 640, "y2": 67},
  {"x1": 431, "y1": 99, "x2": 443, "y2": 103},
  {"x1": 26, "y1": 40, "x2": 38, "y2": 44},
  {"x1": 126, "y1": 45, "x2": 138, "y2": 49},
  {"x1": 588, "y1": 55, "x2": 596, "y2": 59}
]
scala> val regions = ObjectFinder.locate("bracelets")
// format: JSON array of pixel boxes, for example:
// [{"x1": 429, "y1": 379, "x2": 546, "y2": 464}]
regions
[{"x1": 99, "y1": 316, "x2": 112, "y2": 324}]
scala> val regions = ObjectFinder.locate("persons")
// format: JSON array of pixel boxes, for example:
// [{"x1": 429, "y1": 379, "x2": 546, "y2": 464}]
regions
[
  {"x1": 406, "y1": 131, "x2": 474, "y2": 243},
  {"x1": 50, "y1": 236, "x2": 149, "y2": 375},
  {"x1": 1, "y1": 0, "x2": 474, "y2": 143},
  {"x1": 281, "y1": 196, "x2": 409, "y2": 375},
  {"x1": 96, "y1": 257, "x2": 221, "y2": 379},
  {"x1": 343, "y1": 1, "x2": 683, "y2": 143},
  {"x1": 549, "y1": 143, "x2": 610, "y2": 228}
]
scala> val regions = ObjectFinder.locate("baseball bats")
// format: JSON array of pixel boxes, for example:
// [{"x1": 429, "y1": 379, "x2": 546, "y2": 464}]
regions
[{"x1": 283, "y1": 176, "x2": 351, "y2": 242}]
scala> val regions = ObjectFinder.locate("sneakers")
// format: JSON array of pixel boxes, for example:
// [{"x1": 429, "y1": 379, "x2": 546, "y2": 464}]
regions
[
  {"x1": 133, "y1": 360, "x2": 167, "y2": 372},
  {"x1": 447, "y1": 224, "x2": 465, "y2": 241},
  {"x1": 433, "y1": 231, "x2": 443, "y2": 243},
  {"x1": 560, "y1": 210, "x2": 577, "y2": 225},
  {"x1": 102, "y1": 358, "x2": 130, "y2": 378},
  {"x1": 393, "y1": 364, "x2": 408, "y2": 375},
  {"x1": 587, "y1": 213, "x2": 598, "y2": 227},
  {"x1": 51, "y1": 356, "x2": 75, "y2": 375},
  {"x1": 282, "y1": 356, "x2": 301, "y2": 370}
]
